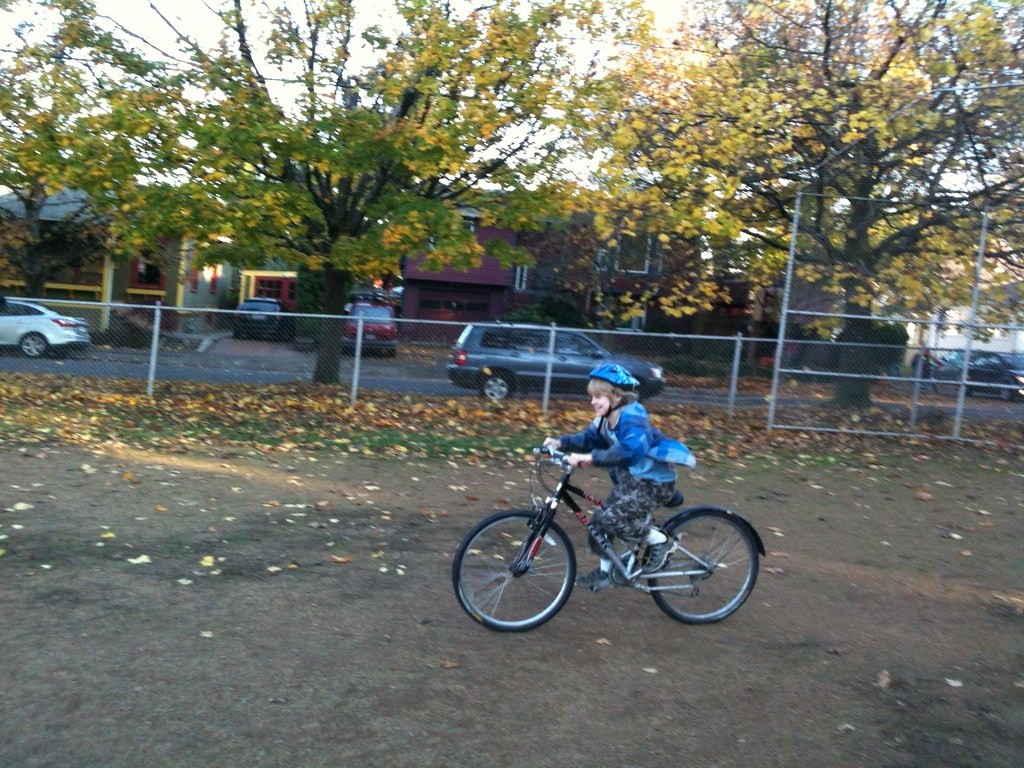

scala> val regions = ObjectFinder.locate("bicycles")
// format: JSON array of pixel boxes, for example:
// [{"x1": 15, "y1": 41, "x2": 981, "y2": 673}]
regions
[{"x1": 450, "y1": 445, "x2": 768, "y2": 632}]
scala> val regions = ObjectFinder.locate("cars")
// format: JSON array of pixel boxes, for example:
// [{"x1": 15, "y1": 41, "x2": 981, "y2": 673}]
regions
[
  {"x1": 340, "y1": 299, "x2": 398, "y2": 356},
  {"x1": 0, "y1": 301, "x2": 92, "y2": 359}
]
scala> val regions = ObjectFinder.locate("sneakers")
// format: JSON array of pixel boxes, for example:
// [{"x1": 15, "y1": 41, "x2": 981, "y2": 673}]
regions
[
  {"x1": 643, "y1": 535, "x2": 678, "y2": 573},
  {"x1": 575, "y1": 568, "x2": 608, "y2": 586}
]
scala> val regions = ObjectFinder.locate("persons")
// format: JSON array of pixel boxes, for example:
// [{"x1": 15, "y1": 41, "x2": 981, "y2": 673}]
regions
[
  {"x1": 543, "y1": 361, "x2": 677, "y2": 587},
  {"x1": 912, "y1": 347, "x2": 943, "y2": 392}
]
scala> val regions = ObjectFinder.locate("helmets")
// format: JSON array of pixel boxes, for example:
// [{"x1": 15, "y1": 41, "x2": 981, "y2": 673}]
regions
[{"x1": 589, "y1": 363, "x2": 641, "y2": 391}]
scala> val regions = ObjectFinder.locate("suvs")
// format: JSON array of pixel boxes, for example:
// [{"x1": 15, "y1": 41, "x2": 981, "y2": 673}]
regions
[
  {"x1": 447, "y1": 325, "x2": 668, "y2": 406},
  {"x1": 232, "y1": 298, "x2": 295, "y2": 342},
  {"x1": 933, "y1": 351, "x2": 1023, "y2": 400}
]
